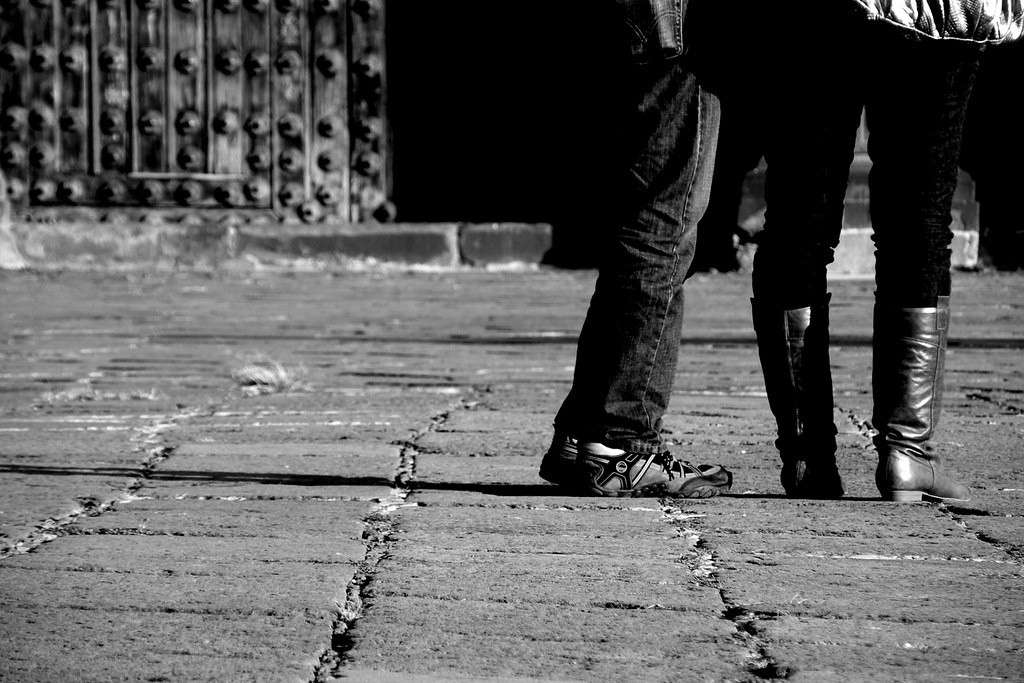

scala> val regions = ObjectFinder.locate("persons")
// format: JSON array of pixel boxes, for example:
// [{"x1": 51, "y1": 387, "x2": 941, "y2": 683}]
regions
[
  {"x1": 537, "y1": 0, "x2": 735, "y2": 499},
  {"x1": 745, "y1": 0, "x2": 1024, "y2": 503}
]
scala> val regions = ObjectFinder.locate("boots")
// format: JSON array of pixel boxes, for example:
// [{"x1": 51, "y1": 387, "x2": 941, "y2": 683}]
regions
[
  {"x1": 871, "y1": 290, "x2": 973, "y2": 502},
  {"x1": 749, "y1": 292, "x2": 848, "y2": 499}
]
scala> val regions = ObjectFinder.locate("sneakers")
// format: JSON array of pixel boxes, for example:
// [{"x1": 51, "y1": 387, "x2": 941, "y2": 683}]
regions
[
  {"x1": 569, "y1": 439, "x2": 732, "y2": 497},
  {"x1": 538, "y1": 422, "x2": 581, "y2": 486}
]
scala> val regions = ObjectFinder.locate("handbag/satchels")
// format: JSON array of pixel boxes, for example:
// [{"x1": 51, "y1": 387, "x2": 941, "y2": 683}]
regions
[{"x1": 850, "y1": 2, "x2": 1022, "y2": 50}]
